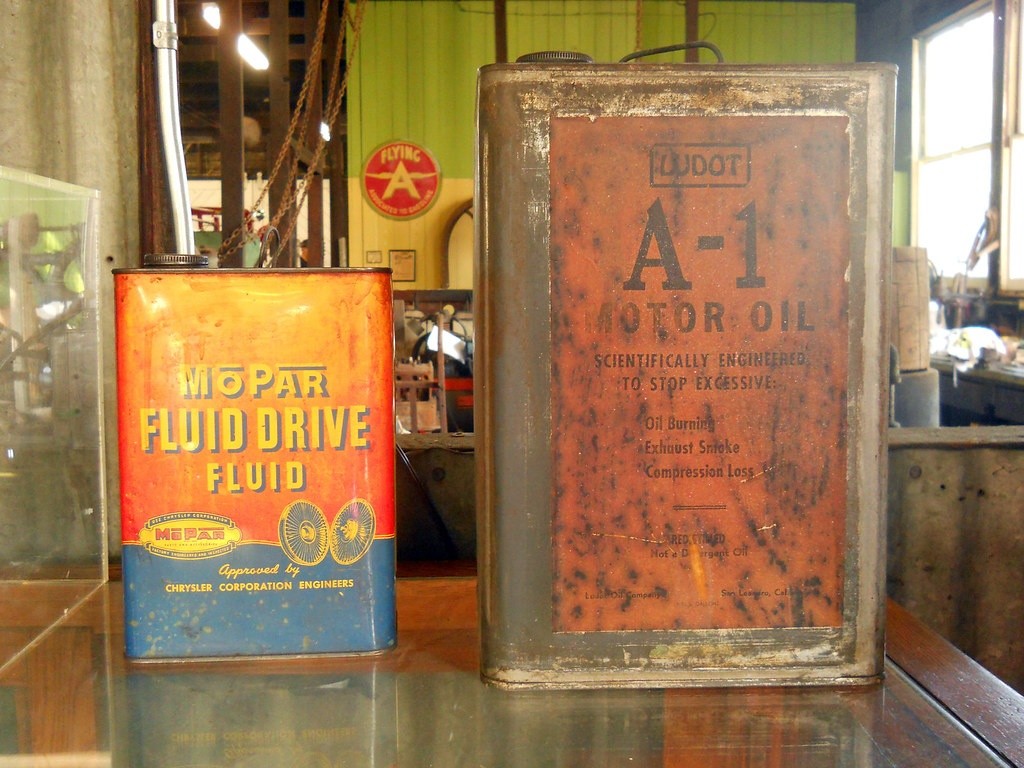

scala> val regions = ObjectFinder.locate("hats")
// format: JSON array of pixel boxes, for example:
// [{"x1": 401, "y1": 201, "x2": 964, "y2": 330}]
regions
[{"x1": 300, "y1": 239, "x2": 308, "y2": 247}]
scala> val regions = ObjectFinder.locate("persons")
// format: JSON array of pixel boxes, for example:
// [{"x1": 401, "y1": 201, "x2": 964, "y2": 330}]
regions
[
  {"x1": 301, "y1": 240, "x2": 308, "y2": 268},
  {"x1": 296, "y1": 239, "x2": 309, "y2": 267}
]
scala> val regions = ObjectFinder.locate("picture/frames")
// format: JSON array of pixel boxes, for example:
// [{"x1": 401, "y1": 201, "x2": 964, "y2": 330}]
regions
[
  {"x1": 366, "y1": 250, "x2": 382, "y2": 263},
  {"x1": 388, "y1": 249, "x2": 417, "y2": 283}
]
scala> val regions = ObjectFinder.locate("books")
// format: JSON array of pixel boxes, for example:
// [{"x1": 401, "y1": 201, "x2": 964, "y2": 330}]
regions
[
  {"x1": 473, "y1": 63, "x2": 892, "y2": 690},
  {"x1": 111, "y1": 267, "x2": 398, "y2": 663}
]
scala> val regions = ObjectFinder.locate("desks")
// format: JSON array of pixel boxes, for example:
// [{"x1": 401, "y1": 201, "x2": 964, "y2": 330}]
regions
[{"x1": 0, "y1": 558, "x2": 1024, "y2": 768}]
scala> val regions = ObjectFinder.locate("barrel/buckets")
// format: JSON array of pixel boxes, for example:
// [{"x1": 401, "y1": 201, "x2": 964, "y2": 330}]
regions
[
  {"x1": 469, "y1": 42, "x2": 896, "y2": 693},
  {"x1": 107, "y1": 250, "x2": 398, "y2": 665}
]
